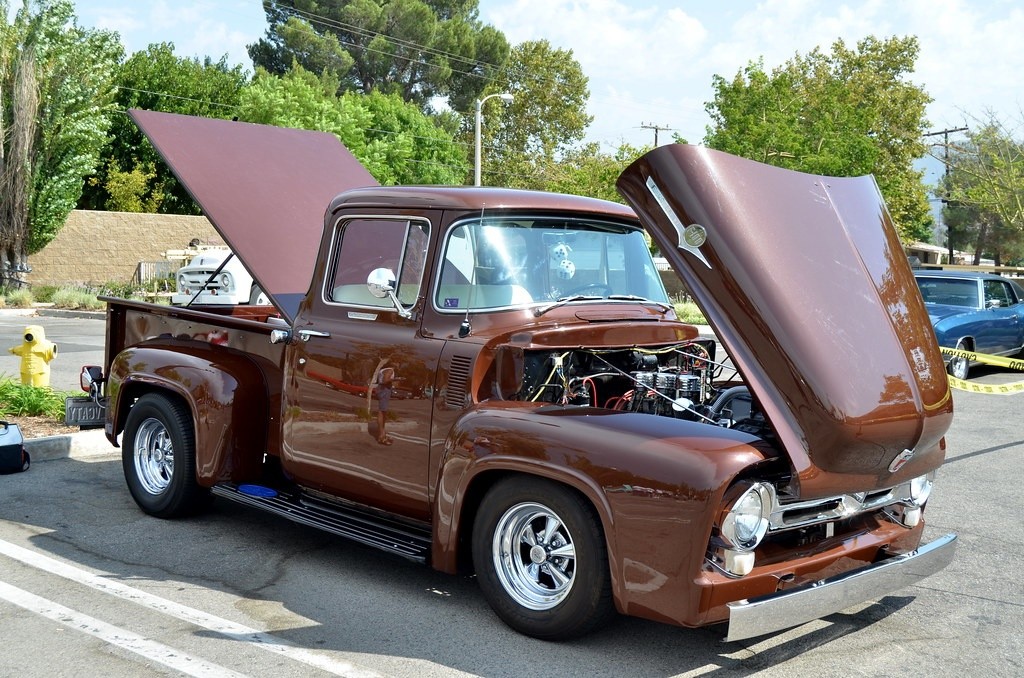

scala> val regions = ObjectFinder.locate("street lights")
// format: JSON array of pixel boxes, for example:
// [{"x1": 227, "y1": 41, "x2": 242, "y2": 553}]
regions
[{"x1": 474, "y1": 93, "x2": 515, "y2": 187}]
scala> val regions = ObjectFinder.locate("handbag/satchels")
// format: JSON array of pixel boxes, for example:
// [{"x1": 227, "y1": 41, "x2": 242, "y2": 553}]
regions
[{"x1": 0, "y1": 421, "x2": 31, "y2": 475}]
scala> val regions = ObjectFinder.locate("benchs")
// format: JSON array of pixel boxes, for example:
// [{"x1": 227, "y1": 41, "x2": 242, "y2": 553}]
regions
[{"x1": 334, "y1": 284, "x2": 544, "y2": 307}]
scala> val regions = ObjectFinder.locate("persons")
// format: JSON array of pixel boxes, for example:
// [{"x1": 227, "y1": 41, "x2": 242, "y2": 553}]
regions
[{"x1": 373, "y1": 360, "x2": 407, "y2": 446}]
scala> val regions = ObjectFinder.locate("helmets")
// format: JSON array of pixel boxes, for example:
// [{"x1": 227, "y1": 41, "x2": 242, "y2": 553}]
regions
[{"x1": 475, "y1": 226, "x2": 527, "y2": 284}]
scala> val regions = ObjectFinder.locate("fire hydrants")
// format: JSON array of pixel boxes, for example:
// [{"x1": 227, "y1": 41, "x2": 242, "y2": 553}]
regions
[{"x1": 8, "y1": 326, "x2": 58, "y2": 390}]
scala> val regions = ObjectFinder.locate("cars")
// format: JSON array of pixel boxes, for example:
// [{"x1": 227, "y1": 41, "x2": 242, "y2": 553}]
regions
[
  {"x1": 911, "y1": 269, "x2": 1024, "y2": 380},
  {"x1": 171, "y1": 248, "x2": 272, "y2": 306},
  {"x1": 64, "y1": 110, "x2": 957, "y2": 643}
]
